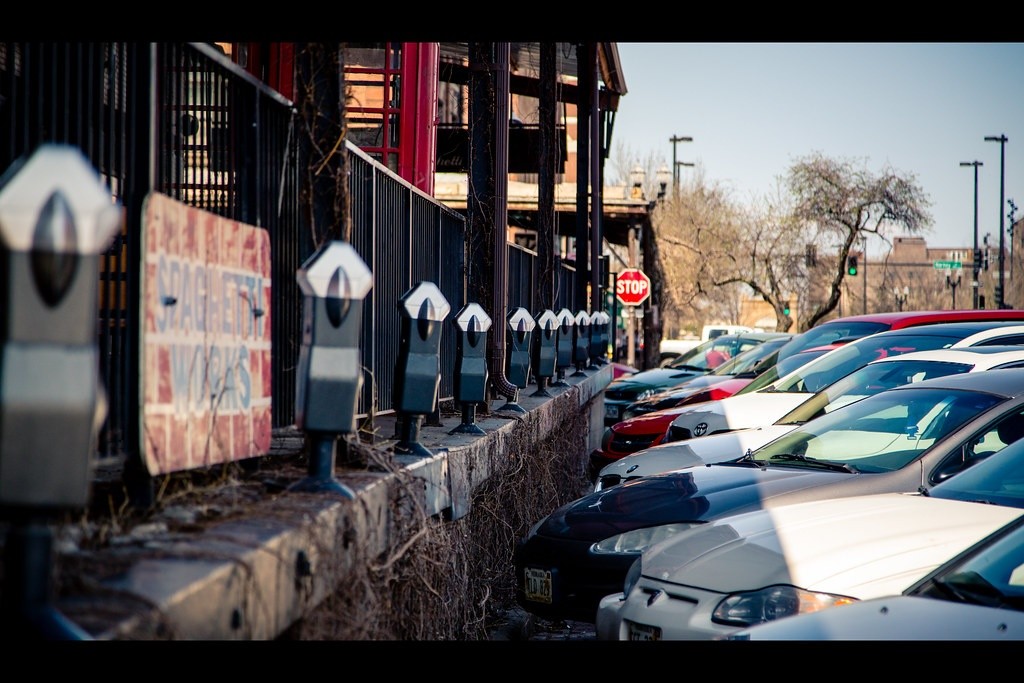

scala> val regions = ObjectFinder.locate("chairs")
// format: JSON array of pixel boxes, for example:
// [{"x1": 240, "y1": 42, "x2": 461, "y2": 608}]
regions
[{"x1": 855, "y1": 398, "x2": 980, "y2": 477}]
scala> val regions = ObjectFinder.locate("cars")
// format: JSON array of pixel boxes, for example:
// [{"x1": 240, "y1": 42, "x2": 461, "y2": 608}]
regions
[{"x1": 513, "y1": 308, "x2": 1024, "y2": 639}]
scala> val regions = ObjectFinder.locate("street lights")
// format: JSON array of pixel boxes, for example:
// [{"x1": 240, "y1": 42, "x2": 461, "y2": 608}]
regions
[
  {"x1": 676, "y1": 161, "x2": 694, "y2": 197},
  {"x1": 960, "y1": 160, "x2": 984, "y2": 310},
  {"x1": 668, "y1": 134, "x2": 694, "y2": 193},
  {"x1": 984, "y1": 131, "x2": 1008, "y2": 308}
]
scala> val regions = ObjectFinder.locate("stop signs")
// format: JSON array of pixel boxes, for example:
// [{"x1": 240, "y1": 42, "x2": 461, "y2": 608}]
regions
[{"x1": 614, "y1": 268, "x2": 653, "y2": 305}]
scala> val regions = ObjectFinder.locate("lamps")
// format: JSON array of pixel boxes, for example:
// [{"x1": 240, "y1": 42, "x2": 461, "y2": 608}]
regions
[
  {"x1": 653, "y1": 158, "x2": 671, "y2": 209},
  {"x1": 632, "y1": 158, "x2": 645, "y2": 199}
]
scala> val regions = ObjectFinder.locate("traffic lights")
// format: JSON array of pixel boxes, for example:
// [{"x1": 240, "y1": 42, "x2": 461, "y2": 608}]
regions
[
  {"x1": 975, "y1": 250, "x2": 983, "y2": 268},
  {"x1": 995, "y1": 286, "x2": 1003, "y2": 304},
  {"x1": 847, "y1": 255, "x2": 858, "y2": 276},
  {"x1": 783, "y1": 302, "x2": 792, "y2": 319}
]
